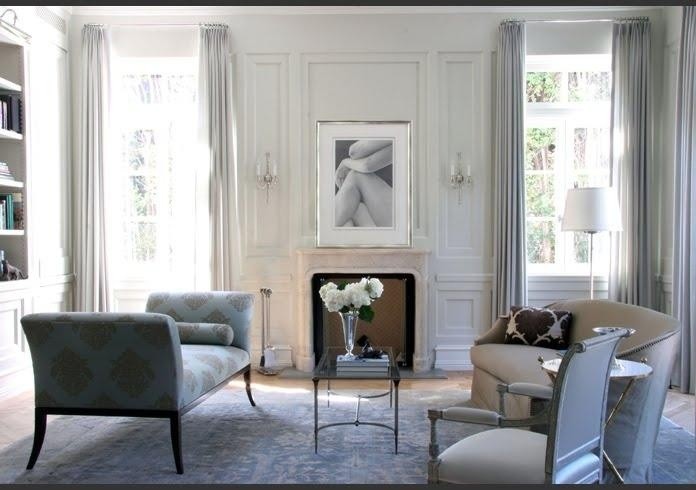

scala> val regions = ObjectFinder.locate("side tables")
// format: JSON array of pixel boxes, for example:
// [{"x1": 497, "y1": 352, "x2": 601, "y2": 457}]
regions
[{"x1": 540, "y1": 358, "x2": 654, "y2": 485}]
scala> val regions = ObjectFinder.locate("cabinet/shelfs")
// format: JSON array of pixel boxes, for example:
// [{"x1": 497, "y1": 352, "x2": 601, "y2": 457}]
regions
[
  {"x1": 0, "y1": 20, "x2": 29, "y2": 282},
  {"x1": 0, "y1": 273, "x2": 74, "y2": 377}
]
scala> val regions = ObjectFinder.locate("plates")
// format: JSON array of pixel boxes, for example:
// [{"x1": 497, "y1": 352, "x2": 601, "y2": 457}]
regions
[
  {"x1": 540, "y1": 357, "x2": 654, "y2": 380},
  {"x1": 593, "y1": 327, "x2": 637, "y2": 337}
]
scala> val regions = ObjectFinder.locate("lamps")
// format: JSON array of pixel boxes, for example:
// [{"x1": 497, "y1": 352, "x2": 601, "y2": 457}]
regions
[{"x1": 562, "y1": 186, "x2": 624, "y2": 299}]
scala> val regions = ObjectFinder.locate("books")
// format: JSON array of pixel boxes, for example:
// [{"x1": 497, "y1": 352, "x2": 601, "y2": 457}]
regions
[
  {"x1": 0, "y1": 193, "x2": 23, "y2": 230},
  {"x1": 336, "y1": 355, "x2": 389, "y2": 373},
  {"x1": 0, "y1": 162, "x2": 15, "y2": 181}
]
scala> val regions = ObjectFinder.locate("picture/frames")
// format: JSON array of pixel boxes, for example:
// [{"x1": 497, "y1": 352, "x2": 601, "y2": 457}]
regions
[{"x1": 314, "y1": 120, "x2": 414, "y2": 249}]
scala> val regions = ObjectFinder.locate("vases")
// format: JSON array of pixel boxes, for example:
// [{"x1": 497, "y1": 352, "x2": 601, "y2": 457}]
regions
[{"x1": 340, "y1": 316, "x2": 358, "y2": 358}]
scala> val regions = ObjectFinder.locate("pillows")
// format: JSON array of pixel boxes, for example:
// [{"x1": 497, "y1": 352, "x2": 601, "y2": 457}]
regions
[{"x1": 505, "y1": 304, "x2": 574, "y2": 351}]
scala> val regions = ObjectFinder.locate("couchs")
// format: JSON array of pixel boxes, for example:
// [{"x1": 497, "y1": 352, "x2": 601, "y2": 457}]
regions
[
  {"x1": 19, "y1": 290, "x2": 255, "y2": 475},
  {"x1": 470, "y1": 298, "x2": 681, "y2": 485}
]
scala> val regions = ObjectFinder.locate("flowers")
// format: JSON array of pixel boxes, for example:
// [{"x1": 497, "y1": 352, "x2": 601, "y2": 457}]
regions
[{"x1": 318, "y1": 278, "x2": 384, "y2": 323}]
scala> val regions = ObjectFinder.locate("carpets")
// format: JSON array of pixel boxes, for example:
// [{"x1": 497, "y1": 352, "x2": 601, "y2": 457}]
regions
[{"x1": 0, "y1": 390, "x2": 694, "y2": 484}]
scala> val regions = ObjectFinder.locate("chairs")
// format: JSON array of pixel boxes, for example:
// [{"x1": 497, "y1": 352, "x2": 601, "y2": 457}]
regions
[{"x1": 426, "y1": 329, "x2": 631, "y2": 484}]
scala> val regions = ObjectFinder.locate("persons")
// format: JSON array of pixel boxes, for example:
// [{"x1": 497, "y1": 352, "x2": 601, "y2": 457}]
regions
[{"x1": 333, "y1": 139, "x2": 392, "y2": 227}]
